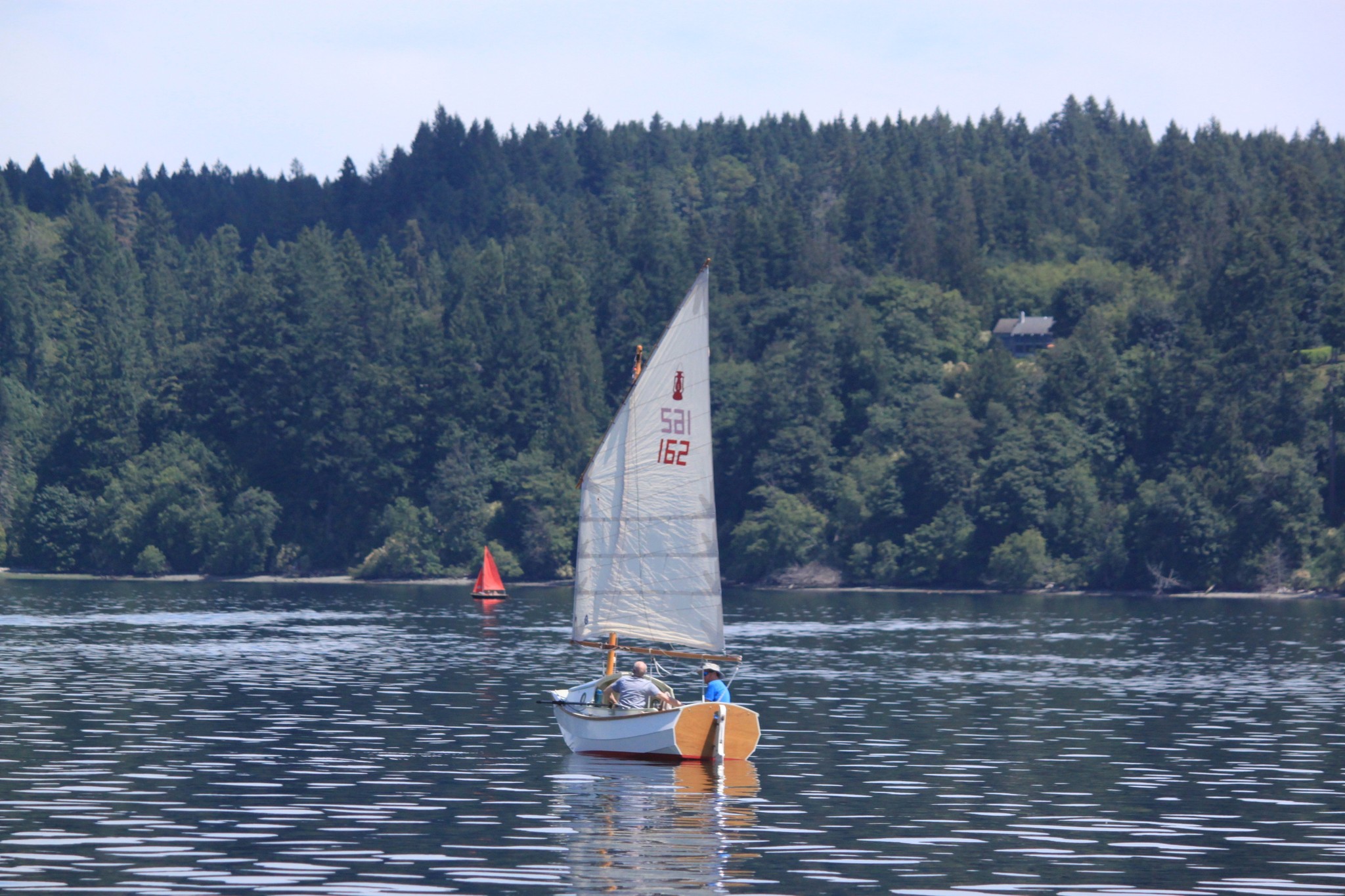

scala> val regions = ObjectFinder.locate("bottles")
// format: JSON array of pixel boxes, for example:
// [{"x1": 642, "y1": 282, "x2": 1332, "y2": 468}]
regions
[{"x1": 595, "y1": 689, "x2": 603, "y2": 707}]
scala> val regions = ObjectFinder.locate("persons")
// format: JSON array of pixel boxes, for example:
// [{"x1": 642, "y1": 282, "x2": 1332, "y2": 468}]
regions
[
  {"x1": 697, "y1": 662, "x2": 731, "y2": 703},
  {"x1": 602, "y1": 661, "x2": 686, "y2": 709}
]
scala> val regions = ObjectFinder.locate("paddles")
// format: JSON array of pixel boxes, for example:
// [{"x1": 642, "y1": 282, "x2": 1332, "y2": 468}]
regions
[{"x1": 535, "y1": 699, "x2": 610, "y2": 707}]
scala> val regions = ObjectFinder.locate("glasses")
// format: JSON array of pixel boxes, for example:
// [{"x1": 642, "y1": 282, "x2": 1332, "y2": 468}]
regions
[{"x1": 704, "y1": 671, "x2": 711, "y2": 676}]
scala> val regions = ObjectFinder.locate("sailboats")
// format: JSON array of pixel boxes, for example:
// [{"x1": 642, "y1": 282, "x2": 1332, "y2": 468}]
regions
[
  {"x1": 471, "y1": 545, "x2": 509, "y2": 600},
  {"x1": 546, "y1": 257, "x2": 763, "y2": 762}
]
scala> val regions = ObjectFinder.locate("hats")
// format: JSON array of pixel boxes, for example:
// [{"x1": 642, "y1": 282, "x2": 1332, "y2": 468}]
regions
[{"x1": 702, "y1": 663, "x2": 725, "y2": 679}]
